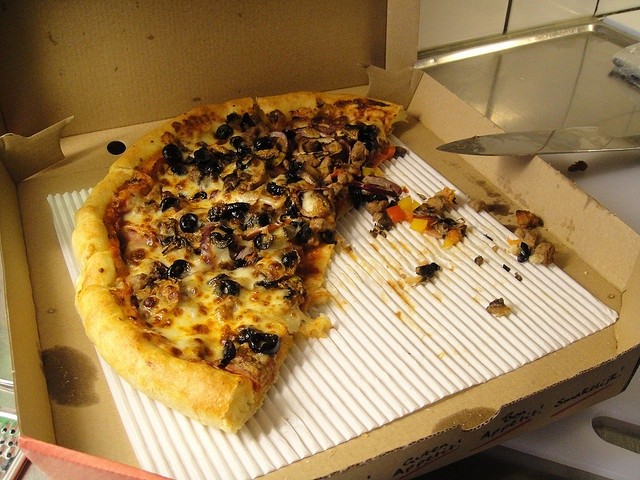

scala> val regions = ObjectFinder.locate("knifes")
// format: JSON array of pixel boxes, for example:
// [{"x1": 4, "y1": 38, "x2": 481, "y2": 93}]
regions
[{"x1": 435, "y1": 120, "x2": 639, "y2": 157}]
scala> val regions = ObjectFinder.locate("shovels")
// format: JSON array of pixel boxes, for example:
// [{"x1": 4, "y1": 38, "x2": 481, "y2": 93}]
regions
[{"x1": 436, "y1": 111, "x2": 640, "y2": 156}]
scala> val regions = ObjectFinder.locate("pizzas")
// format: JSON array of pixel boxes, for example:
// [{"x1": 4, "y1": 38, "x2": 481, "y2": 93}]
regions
[{"x1": 75, "y1": 90, "x2": 407, "y2": 431}]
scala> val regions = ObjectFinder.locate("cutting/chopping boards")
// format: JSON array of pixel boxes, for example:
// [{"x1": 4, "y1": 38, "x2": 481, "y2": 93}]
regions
[{"x1": 496, "y1": 157, "x2": 639, "y2": 480}]
